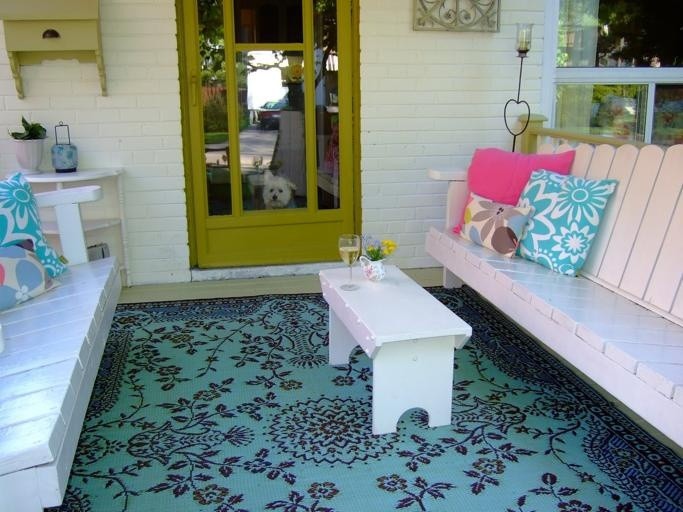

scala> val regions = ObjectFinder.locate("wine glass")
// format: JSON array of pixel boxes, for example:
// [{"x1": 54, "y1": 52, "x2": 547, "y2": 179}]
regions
[
  {"x1": 12, "y1": 138, "x2": 46, "y2": 174},
  {"x1": 336, "y1": 234, "x2": 360, "y2": 290}
]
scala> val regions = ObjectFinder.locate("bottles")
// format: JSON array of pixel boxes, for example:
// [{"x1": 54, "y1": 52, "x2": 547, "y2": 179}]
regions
[{"x1": 49, "y1": 143, "x2": 79, "y2": 173}]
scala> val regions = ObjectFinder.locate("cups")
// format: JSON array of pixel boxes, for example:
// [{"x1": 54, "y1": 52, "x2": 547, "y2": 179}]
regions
[{"x1": 359, "y1": 256, "x2": 388, "y2": 281}]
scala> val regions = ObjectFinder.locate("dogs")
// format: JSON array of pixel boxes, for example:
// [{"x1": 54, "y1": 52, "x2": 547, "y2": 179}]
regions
[{"x1": 262, "y1": 169, "x2": 298, "y2": 210}]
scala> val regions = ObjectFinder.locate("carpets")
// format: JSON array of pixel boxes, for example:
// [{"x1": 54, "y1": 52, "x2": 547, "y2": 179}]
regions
[{"x1": 41, "y1": 284, "x2": 682, "y2": 511}]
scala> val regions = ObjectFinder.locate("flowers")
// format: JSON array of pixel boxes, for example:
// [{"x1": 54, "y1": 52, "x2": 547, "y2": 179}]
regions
[{"x1": 360, "y1": 230, "x2": 399, "y2": 262}]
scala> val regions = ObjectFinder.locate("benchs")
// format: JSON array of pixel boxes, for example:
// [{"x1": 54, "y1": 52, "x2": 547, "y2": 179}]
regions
[
  {"x1": 423, "y1": 140, "x2": 682, "y2": 448},
  {"x1": 0, "y1": 184, "x2": 124, "y2": 511}
]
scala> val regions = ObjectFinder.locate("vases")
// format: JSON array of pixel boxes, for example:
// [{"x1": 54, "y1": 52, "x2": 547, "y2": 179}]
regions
[{"x1": 357, "y1": 255, "x2": 388, "y2": 283}]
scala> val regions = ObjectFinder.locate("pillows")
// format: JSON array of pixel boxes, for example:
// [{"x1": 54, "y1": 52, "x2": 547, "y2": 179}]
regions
[
  {"x1": 0, "y1": 235, "x2": 63, "y2": 313},
  {"x1": 511, "y1": 166, "x2": 620, "y2": 280},
  {"x1": 0, "y1": 168, "x2": 71, "y2": 279},
  {"x1": 453, "y1": 145, "x2": 577, "y2": 236},
  {"x1": 458, "y1": 188, "x2": 533, "y2": 261}
]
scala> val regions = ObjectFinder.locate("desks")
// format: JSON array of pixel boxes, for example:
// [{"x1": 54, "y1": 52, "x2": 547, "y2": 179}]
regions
[{"x1": 318, "y1": 263, "x2": 475, "y2": 436}]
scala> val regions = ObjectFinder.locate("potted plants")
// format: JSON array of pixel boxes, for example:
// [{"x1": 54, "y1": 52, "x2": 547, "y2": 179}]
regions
[{"x1": 6, "y1": 114, "x2": 50, "y2": 175}]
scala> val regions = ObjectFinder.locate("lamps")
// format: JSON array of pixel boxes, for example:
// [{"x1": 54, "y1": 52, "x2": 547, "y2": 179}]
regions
[{"x1": 502, "y1": 20, "x2": 534, "y2": 153}]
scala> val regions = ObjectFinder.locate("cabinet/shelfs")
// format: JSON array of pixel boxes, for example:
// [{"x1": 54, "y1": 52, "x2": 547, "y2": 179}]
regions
[{"x1": 6, "y1": 164, "x2": 131, "y2": 236}]
